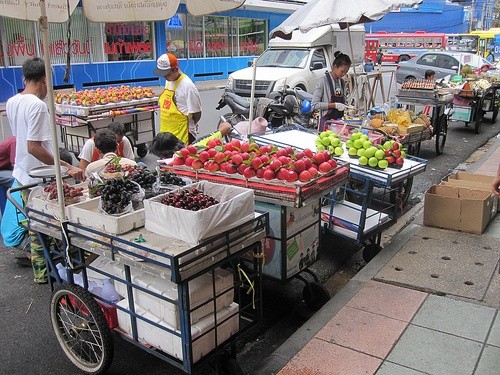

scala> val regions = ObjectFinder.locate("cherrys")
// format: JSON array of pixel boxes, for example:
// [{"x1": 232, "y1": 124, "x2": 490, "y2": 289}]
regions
[{"x1": 161, "y1": 188, "x2": 220, "y2": 211}]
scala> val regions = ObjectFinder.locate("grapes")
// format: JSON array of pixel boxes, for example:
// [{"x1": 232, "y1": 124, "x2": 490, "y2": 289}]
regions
[
  {"x1": 44, "y1": 180, "x2": 84, "y2": 206},
  {"x1": 100, "y1": 162, "x2": 187, "y2": 216}
]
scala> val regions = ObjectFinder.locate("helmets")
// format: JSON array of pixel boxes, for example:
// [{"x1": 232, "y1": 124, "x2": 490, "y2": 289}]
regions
[{"x1": 300, "y1": 99, "x2": 313, "y2": 114}]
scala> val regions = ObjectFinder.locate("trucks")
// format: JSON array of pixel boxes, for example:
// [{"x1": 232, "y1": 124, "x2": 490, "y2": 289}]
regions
[{"x1": 224, "y1": 23, "x2": 366, "y2": 118}]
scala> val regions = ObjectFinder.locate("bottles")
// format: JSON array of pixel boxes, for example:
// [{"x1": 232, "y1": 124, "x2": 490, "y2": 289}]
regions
[{"x1": 55, "y1": 262, "x2": 119, "y2": 303}]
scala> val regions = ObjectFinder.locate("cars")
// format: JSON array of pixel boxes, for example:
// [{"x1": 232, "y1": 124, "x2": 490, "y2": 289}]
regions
[{"x1": 396, "y1": 52, "x2": 498, "y2": 85}]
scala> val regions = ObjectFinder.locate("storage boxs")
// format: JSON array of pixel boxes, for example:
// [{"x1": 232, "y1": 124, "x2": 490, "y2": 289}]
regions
[
  {"x1": 423, "y1": 170, "x2": 500, "y2": 235},
  {"x1": 143, "y1": 180, "x2": 255, "y2": 246},
  {"x1": 31, "y1": 181, "x2": 91, "y2": 218},
  {"x1": 116, "y1": 298, "x2": 239, "y2": 364},
  {"x1": 113, "y1": 262, "x2": 235, "y2": 329},
  {"x1": 226, "y1": 198, "x2": 319, "y2": 281},
  {"x1": 66, "y1": 195, "x2": 146, "y2": 236}
]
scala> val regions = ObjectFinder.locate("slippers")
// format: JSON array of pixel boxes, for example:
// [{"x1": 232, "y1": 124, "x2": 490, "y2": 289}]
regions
[{"x1": 13, "y1": 256, "x2": 33, "y2": 266}]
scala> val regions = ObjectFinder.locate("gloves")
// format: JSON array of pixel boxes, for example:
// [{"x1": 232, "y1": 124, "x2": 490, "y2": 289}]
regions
[{"x1": 335, "y1": 102, "x2": 350, "y2": 111}]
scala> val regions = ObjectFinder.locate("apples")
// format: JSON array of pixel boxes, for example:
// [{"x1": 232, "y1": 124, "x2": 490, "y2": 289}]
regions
[{"x1": 316, "y1": 130, "x2": 405, "y2": 168}]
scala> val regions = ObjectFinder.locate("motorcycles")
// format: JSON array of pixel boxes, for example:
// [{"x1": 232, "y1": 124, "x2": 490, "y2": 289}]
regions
[{"x1": 216, "y1": 85, "x2": 311, "y2": 134}]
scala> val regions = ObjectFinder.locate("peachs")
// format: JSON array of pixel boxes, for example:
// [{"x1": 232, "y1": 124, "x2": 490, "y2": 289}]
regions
[
  {"x1": 55, "y1": 85, "x2": 154, "y2": 105},
  {"x1": 168, "y1": 138, "x2": 338, "y2": 182}
]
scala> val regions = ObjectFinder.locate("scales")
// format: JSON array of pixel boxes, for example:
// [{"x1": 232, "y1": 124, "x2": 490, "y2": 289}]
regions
[{"x1": 24, "y1": 165, "x2": 91, "y2": 221}]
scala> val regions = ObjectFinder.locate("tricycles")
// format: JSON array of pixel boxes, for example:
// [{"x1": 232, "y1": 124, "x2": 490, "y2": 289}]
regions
[{"x1": 366, "y1": 85, "x2": 500, "y2": 157}]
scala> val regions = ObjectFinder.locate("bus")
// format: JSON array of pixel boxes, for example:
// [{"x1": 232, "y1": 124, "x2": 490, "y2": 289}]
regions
[
  {"x1": 365, "y1": 30, "x2": 448, "y2": 64},
  {"x1": 448, "y1": 28, "x2": 500, "y2": 62}
]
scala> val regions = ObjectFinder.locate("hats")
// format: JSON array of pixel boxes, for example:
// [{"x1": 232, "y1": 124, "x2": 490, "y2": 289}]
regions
[
  {"x1": 153, "y1": 53, "x2": 178, "y2": 77},
  {"x1": 235, "y1": 117, "x2": 273, "y2": 137},
  {"x1": 481, "y1": 63, "x2": 489, "y2": 69}
]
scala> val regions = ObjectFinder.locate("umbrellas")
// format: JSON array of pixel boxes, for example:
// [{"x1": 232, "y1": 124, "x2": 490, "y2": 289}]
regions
[
  {"x1": 0, "y1": 1, "x2": 248, "y2": 284},
  {"x1": 269, "y1": 0, "x2": 423, "y2": 117}
]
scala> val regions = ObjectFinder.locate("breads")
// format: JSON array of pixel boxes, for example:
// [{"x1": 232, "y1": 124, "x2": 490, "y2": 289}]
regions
[{"x1": 371, "y1": 113, "x2": 432, "y2": 141}]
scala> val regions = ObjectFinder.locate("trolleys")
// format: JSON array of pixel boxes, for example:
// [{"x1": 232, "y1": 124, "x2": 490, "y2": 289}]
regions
[
  {"x1": 55, "y1": 96, "x2": 160, "y2": 158},
  {"x1": 6, "y1": 121, "x2": 428, "y2": 375}
]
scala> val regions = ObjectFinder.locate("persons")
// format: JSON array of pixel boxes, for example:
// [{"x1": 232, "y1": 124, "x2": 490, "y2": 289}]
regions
[
  {"x1": 153, "y1": 53, "x2": 203, "y2": 147},
  {"x1": 0, "y1": 57, "x2": 230, "y2": 285},
  {"x1": 376, "y1": 50, "x2": 384, "y2": 69},
  {"x1": 425, "y1": 70, "x2": 434, "y2": 81},
  {"x1": 311, "y1": 51, "x2": 352, "y2": 133}
]
